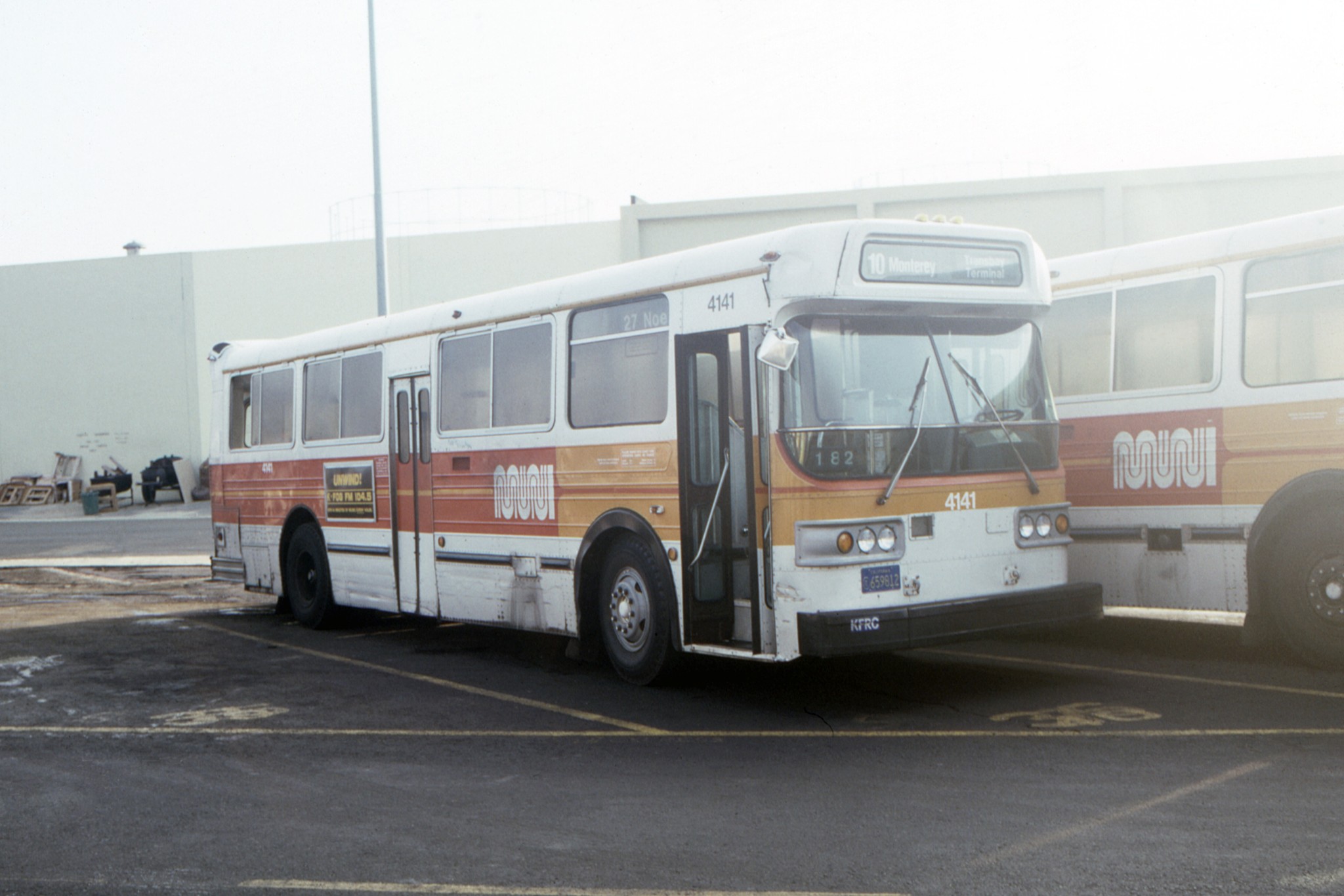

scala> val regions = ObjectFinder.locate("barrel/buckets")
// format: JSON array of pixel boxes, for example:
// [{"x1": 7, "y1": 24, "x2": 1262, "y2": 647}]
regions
[{"x1": 80, "y1": 491, "x2": 99, "y2": 515}]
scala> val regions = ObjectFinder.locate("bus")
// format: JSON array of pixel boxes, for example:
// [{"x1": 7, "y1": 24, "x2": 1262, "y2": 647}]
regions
[
  {"x1": 1037, "y1": 207, "x2": 1344, "y2": 670},
  {"x1": 205, "y1": 219, "x2": 1104, "y2": 685}
]
